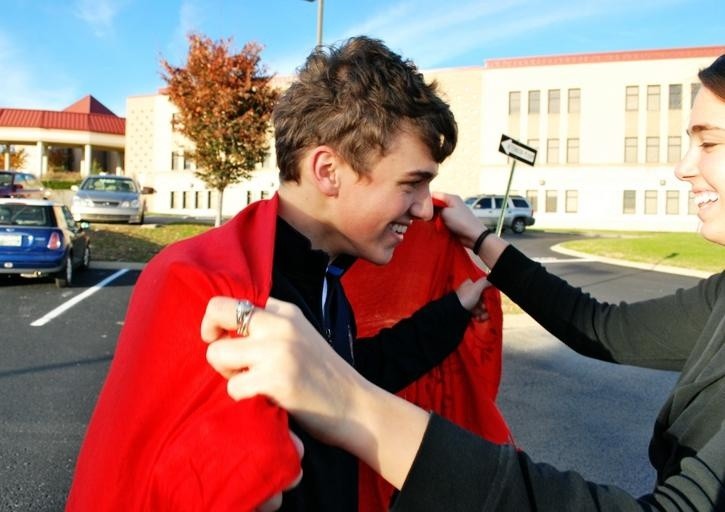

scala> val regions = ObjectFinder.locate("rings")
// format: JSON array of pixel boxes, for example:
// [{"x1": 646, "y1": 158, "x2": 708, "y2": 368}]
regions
[{"x1": 235, "y1": 296, "x2": 255, "y2": 336}]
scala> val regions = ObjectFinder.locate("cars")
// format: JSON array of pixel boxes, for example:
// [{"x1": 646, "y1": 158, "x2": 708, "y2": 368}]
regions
[
  {"x1": 0, "y1": 171, "x2": 154, "y2": 289},
  {"x1": 463, "y1": 195, "x2": 535, "y2": 234}
]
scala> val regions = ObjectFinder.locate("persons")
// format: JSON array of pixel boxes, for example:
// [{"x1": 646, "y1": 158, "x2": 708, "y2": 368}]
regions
[
  {"x1": 200, "y1": 52, "x2": 725, "y2": 512},
  {"x1": 62, "y1": 36, "x2": 495, "y2": 512}
]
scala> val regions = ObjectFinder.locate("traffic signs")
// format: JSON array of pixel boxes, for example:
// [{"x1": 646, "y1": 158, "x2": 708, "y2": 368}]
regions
[{"x1": 497, "y1": 133, "x2": 539, "y2": 166}]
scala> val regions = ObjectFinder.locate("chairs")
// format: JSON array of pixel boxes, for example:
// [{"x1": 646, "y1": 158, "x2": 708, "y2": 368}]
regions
[
  {"x1": 94, "y1": 180, "x2": 129, "y2": 191},
  {"x1": 1, "y1": 208, "x2": 13, "y2": 225}
]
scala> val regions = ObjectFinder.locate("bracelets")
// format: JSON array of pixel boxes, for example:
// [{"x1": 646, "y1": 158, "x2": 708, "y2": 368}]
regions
[{"x1": 472, "y1": 227, "x2": 496, "y2": 255}]
